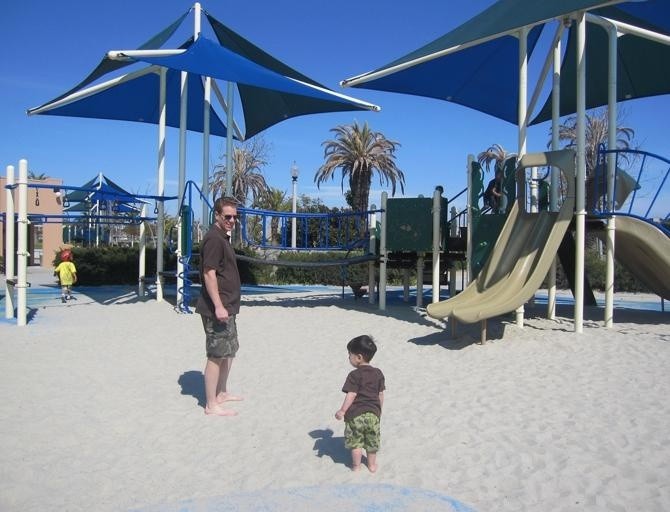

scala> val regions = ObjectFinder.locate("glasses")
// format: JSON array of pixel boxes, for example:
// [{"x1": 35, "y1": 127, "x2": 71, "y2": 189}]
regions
[{"x1": 220, "y1": 213, "x2": 238, "y2": 220}]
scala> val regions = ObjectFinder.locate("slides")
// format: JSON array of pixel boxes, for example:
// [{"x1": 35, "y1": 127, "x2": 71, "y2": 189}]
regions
[
  {"x1": 427, "y1": 199, "x2": 577, "y2": 325},
  {"x1": 588, "y1": 216, "x2": 670, "y2": 302}
]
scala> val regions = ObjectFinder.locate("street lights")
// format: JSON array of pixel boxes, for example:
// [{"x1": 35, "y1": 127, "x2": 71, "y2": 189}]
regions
[{"x1": 291, "y1": 161, "x2": 299, "y2": 251}]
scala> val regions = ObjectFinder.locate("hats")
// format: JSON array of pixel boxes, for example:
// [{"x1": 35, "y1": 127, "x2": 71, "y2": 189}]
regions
[{"x1": 61, "y1": 251, "x2": 74, "y2": 261}]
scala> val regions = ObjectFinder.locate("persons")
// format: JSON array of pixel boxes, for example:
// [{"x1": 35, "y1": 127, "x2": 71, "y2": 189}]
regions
[
  {"x1": 485, "y1": 170, "x2": 503, "y2": 213},
  {"x1": 195, "y1": 197, "x2": 243, "y2": 416},
  {"x1": 54, "y1": 249, "x2": 77, "y2": 303},
  {"x1": 436, "y1": 185, "x2": 448, "y2": 250},
  {"x1": 334, "y1": 335, "x2": 386, "y2": 474}
]
